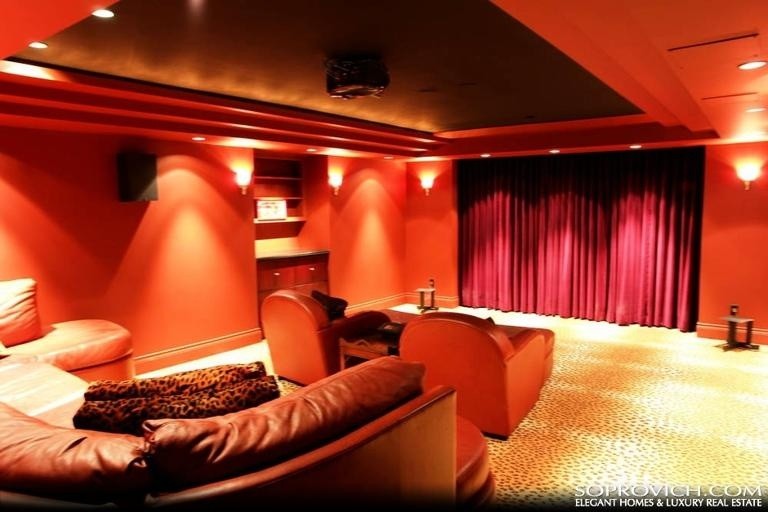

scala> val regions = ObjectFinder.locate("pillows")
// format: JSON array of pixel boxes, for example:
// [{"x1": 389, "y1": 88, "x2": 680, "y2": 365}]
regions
[{"x1": 74, "y1": 362, "x2": 278, "y2": 435}]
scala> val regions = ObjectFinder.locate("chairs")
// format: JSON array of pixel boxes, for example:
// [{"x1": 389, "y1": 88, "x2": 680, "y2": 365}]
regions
[
  {"x1": 397, "y1": 312, "x2": 555, "y2": 438},
  {"x1": 260, "y1": 290, "x2": 392, "y2": 385}
]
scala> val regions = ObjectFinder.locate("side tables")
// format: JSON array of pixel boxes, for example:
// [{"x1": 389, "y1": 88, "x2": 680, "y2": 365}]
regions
[{"x1": 338, "y1": 336, "x2": 397, "y2": 372}]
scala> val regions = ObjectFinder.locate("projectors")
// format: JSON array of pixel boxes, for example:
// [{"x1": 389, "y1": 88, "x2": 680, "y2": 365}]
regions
[{"x1": 328, "y1": 58, "x2": 390, "y2": 101}]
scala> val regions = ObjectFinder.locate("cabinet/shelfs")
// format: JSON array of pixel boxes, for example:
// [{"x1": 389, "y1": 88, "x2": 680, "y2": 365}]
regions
[
  {"x1": 253, "y1": 156, "x2": 306, "y2": 224},
  {"x1": 257, "y1": 254, "x2": 329, "y2": 339}
]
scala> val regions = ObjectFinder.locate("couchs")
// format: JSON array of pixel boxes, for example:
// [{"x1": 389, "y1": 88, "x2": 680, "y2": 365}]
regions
[{"x1": 0, "y1": 277, "x2": 495, "y2": 511}]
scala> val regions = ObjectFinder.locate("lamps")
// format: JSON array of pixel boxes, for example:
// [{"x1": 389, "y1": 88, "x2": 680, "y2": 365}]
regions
[
  {"x1": 421, "y1": 178, "x2": 433, "y2": 196},
  {"x1": 235, "y1": 173, "x2": 251, "y2": 195},
  {"x1": 328, "y1": 175, "x2": 343, "y2": 195},
  {"x1": 736, "y1": 165, "x2": 761, "y2": 189},
  {"x1": 325, "y1": 53, "x2": 389, "y2": 99}
]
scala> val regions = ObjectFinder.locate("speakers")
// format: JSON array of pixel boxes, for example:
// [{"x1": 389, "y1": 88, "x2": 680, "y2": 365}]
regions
[{"x1": 117, "y1": 151, "x2": 159, "y2": 202}]
